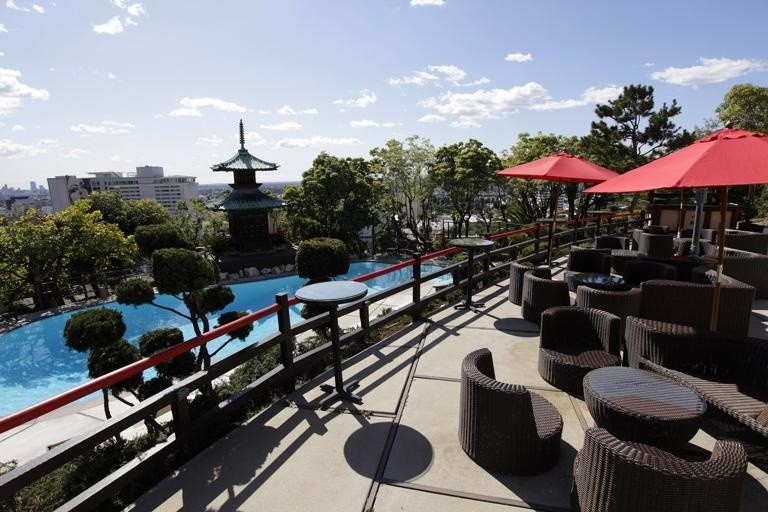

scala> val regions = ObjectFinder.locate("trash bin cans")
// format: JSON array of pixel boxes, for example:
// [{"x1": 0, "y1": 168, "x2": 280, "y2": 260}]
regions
[{"x1": 99, "y1": 287, "x2": 106, "y2": 298}]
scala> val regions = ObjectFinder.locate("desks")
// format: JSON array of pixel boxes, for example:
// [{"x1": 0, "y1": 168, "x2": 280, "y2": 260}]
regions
[
  {"x1": 295, "y1": 281, "x2": 368, "y2": 411},
  {"x1": 582, "y1": 365, "x2": 708, "y2": 443},
  {"x1": 446, "y1": 237, "x2": 494, "y2": 314}
]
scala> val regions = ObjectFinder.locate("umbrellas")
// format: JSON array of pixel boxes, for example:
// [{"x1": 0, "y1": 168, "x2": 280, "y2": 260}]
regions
[
  {"x1": 496, "y1": 147, "x2": 620, "y2": 264},
  {"x1": 583, "y1": 120, "x2": 768, "y2": 332}
]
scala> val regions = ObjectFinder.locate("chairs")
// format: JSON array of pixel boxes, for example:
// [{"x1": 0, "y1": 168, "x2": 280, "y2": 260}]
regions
[
  {"x1": 459, "y1": 347, "x2": 564, "y2": 472},
  {"x1": 499, "y1": 220, "x2": 766, "y2": 390},
  {"x1": 626, "y1": 308, "x2": 767, "y2": 457},
  {"x1": 569, "y1": 429, "x2": 748, "y2": 512}
]
